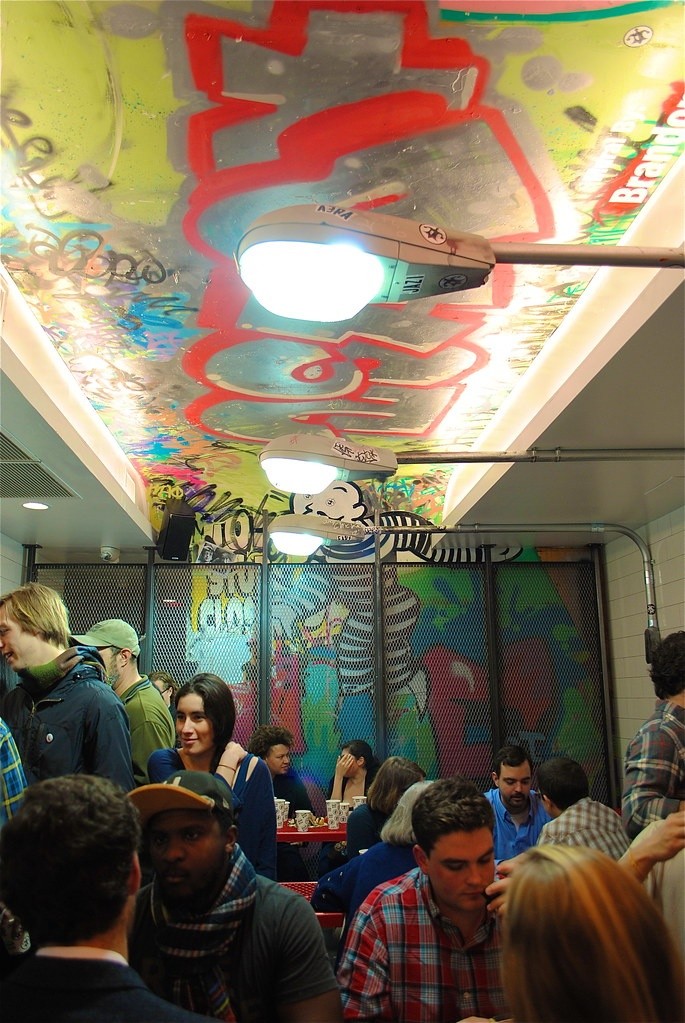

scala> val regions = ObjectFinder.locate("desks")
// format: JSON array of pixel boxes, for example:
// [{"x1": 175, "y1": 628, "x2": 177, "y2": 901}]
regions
[{"x1": 276, "y1": 819, "x2": 347, "y2": 841}]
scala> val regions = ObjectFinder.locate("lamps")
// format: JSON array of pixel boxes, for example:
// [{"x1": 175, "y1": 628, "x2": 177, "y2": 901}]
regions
[
  {"x1": 268, "y1": 515, "x2": 660, "y2": 664},
  {"x1": 258, "y1": 434, "x2": 685, "y2": 495},
  {"x1": 236, "y1": 205, "x2": 685, "y2": 322}
]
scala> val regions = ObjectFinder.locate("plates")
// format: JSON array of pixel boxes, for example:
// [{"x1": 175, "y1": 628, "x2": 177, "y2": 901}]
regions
[{"x1": 309, "y1": 822, "x2": 328, "y2": 828}]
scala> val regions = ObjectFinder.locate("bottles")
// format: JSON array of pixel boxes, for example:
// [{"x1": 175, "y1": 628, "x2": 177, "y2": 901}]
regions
[{"x1": 0, "y1": 903, "x2": 32, "y2": 956}]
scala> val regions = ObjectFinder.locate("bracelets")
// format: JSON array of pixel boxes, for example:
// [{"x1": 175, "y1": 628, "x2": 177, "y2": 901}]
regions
[
  {"x1": 218, "y1": 765, "x2": 236, "y2": 773},
  {"x1": 627, "y1": 851, "x2": 648, "y2": 879}
]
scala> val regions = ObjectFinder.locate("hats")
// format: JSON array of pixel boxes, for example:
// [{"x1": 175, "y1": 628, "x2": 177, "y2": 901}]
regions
[
  {"x1": 126, "y1": 771, "x2": 234, "y2": 825},
  {"x1": 68, "y1": 619, "x2": 140, "y2": 658}
]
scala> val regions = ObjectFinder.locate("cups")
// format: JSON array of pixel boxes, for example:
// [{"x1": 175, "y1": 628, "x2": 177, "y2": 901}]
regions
[
  {"x1": 352, "y1": 796, "x2": 368, "y2": 810},
  {"x1": 295, "y1": 810, "x2": 311, "y2": 832},
  {"x1": 274, "y1": 796, "x2": 290, "y2": 829},
  {"x1": 325, "y1": 799, "x2": 341, "y2": 830},
  {"x1": 340, "y1": 803, "x2": 350, "y2": 824},
  {"x1": 359, "y1": 849, "x2": 368, "y2": 855}
]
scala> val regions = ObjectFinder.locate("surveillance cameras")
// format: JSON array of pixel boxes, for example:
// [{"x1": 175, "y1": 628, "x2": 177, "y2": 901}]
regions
[{"x1": 100, "y1": 546, "x2": 120, "y2": 563}]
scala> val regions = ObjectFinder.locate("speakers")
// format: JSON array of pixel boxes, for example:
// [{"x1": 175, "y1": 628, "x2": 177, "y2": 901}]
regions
[{"x1": 156, "y1": 498, "x2": 196, "y2": 561}]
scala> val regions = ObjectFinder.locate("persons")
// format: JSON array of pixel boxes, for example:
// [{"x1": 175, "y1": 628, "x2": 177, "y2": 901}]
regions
[
  {"x1": 247, "y1": 725, "x2": 316, "y2": 882},
  {"x1": 70, "y1": 619, "x2": 175, "y2": 788},
  {"x1": 336, "y1": 777, "x2": 517, "y2": 1023},
  {"x1": 498, "y1": 843, "x2": 685, "y2": 1023},
  {"x1": 0, "y1": 717, "x2": 31, "y2": 954},
  {"x1": 147, "y1": 674, "x2": 276, "y2": 882},
  {"x1": 148, "y1": 672, "x2": 182, "y2": 748},
  {"x1": 622, "y1": 631, "x2": 685, "y2": 841},
  {"x1": 322, "y1": 741, "x2": 381, "y2": 872},
  {"x1": 483, "y1": 746, "x2": 554, "y2": 866},
  {"x1": 0, "y1": 774, "x2": 228, "y2": 1023},
  {"x1": 346, "y1": 756, "x2": 426, "y2": 861},
  {"x1": 126, "y1": 770, "x2": 343, "y2": 1023},
  {"x1": 0, "y1": 582, "x2": 135, "y2": 792},
  {"x1": 310, "y1": 780, "x2": 436, "y2": 977},
  {"x1": 537, "y1": 757, "x2": 632, "y2": 860},
  {"x1": 617, "y1": 811, "x2": 685, "y2": 971}
]
